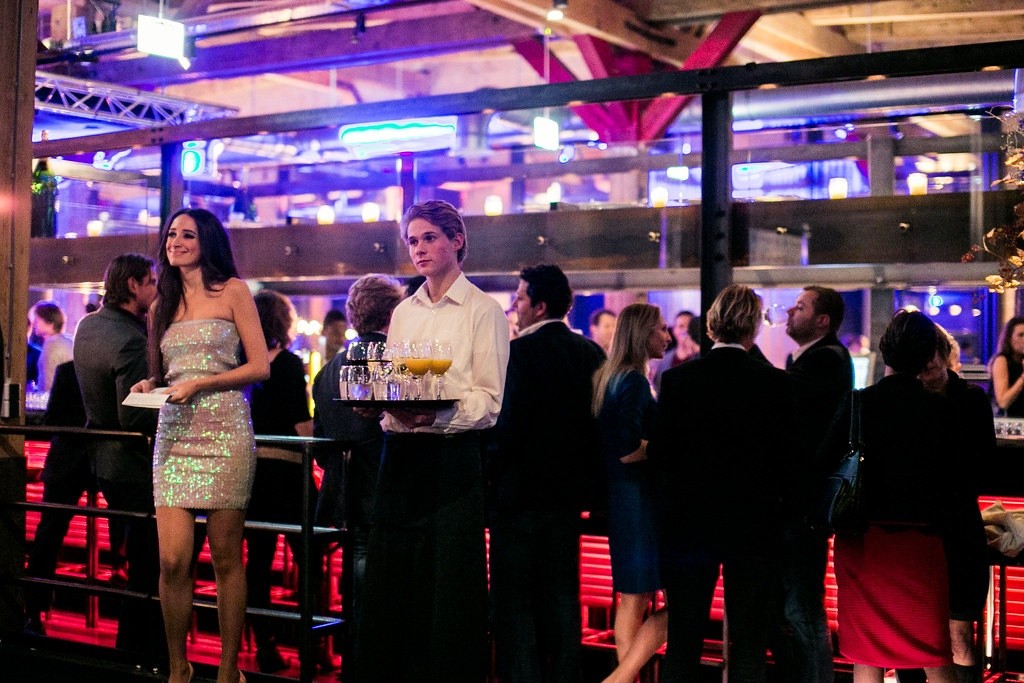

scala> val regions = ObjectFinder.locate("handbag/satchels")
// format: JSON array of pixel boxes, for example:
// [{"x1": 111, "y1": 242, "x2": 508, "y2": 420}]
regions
[{"x1": 819, "y1": 389, "x2": 873, "y2": 538}]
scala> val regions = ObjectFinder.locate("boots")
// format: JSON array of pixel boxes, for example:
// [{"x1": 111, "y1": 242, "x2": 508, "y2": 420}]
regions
[
  {"x1": 299, "y1": 568, "x2": 336, "y2": 679},
  {"x1": 249, "y1": 569, "x2": 293, "y2": 674}
]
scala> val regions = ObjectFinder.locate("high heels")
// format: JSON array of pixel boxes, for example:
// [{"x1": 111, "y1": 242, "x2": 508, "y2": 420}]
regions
[
  {"x1": 216, "y1": 669, "x2": 246, "y2": 683},
  {"x1": 168, "y1": 660, "x2": 193, "y2": 683}
]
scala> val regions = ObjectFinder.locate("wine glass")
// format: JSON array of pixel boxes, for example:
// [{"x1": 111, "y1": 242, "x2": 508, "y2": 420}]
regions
[{"x1": 391, "y1": 340, "x2": 453, "y2": 400}]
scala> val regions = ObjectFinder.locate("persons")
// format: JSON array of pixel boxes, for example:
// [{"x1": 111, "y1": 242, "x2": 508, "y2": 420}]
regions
[
  {"x1": 130, "y1": 208, "x2": 271, "y2": 683},
  {"x1": 20, "y1": 252, "x2": 170, "y2": 659},
  {"x1": 353, "y1": 200, "x2": 512, "y2": 683},
  {"x1": 489, "y1": 266, "x2": 606, "y2": 683},
  {"x1": 236, "y1": 272, "x2": 428, "y2": 683},
  {"x1": 590, "y1": 287, "x2": 1024, "y2": 683}
]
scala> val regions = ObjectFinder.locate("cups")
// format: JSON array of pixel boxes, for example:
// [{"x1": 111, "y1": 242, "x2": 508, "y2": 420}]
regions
[
  {"x1": 651, "y1": 187, "x2": 668, "y2": 208},
  {"x1": 317, "y1": 205, "x2": 334, "y2": 225},
  {"x1": 829, "y1": 177, "x2": 848, "y2": 200},
  {"x1": 485, "y1": 196, "x2": 502, "y2": 216},
  {"x1": 906, "y1": 173, "x2": 928, "y2": 195},
  {"x1": 362, "y1": 202, "x2": 378, "y2": 222},
  {"x1": 337, "y1": 341, "x2": 391, "y2": 400},
  {"x1": 87, "y1": 219, "x2": 102, "y2": 237}
]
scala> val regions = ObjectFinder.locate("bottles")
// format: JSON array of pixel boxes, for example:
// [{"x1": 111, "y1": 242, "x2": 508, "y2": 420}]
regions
[{"x1": 30, "y1": 129, "x2": 60, "y2": 237}]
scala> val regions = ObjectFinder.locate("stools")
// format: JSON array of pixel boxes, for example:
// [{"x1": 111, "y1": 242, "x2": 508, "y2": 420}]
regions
[{"x1": 978, "y1": 547, "x2": 1024, "y2": 673}]
